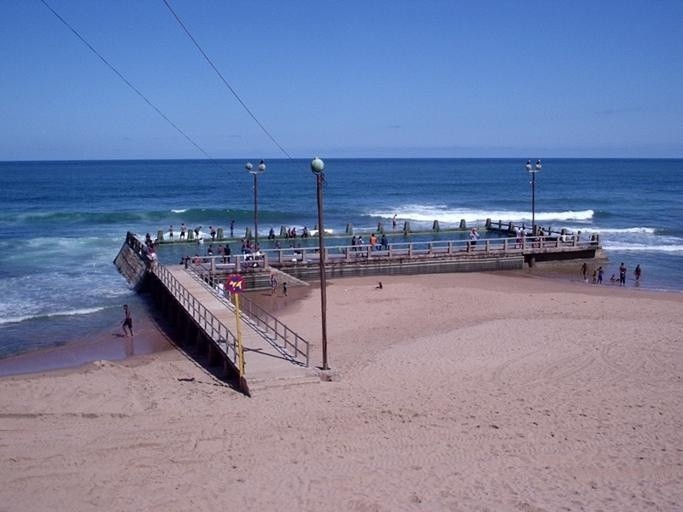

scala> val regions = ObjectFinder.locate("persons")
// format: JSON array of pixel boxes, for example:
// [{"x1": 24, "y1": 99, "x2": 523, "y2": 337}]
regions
[
  {"x1": 121, "y1": 305, "x2": 133, "y2": 337},
  {"x1": 514, "y1": 225, "x2": 546, "y2": 248},
  {"x1": 469, "y1": 228, "x2": 480, "y2": 251},
  {"x1": 633, "y1": 264, "x2": 641, "y2": 280},
  {"x1": 581, "y1": 261, "x2": 627, "y2": 286},
  {"x1": 145, "y1": 214, "x2": 397, "y2": 295}
]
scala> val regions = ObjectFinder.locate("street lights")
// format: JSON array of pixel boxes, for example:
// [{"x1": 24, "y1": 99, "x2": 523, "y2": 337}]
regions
[
  {"x1": 307, "y1": 153, "x2": 332, "y2": 368},
  {"x1": 522, "y1": 158, "x2": 542, "y2": 236},
  {"x1": 244, "y1": 159, "x2": 266, "y2": 250}
]
